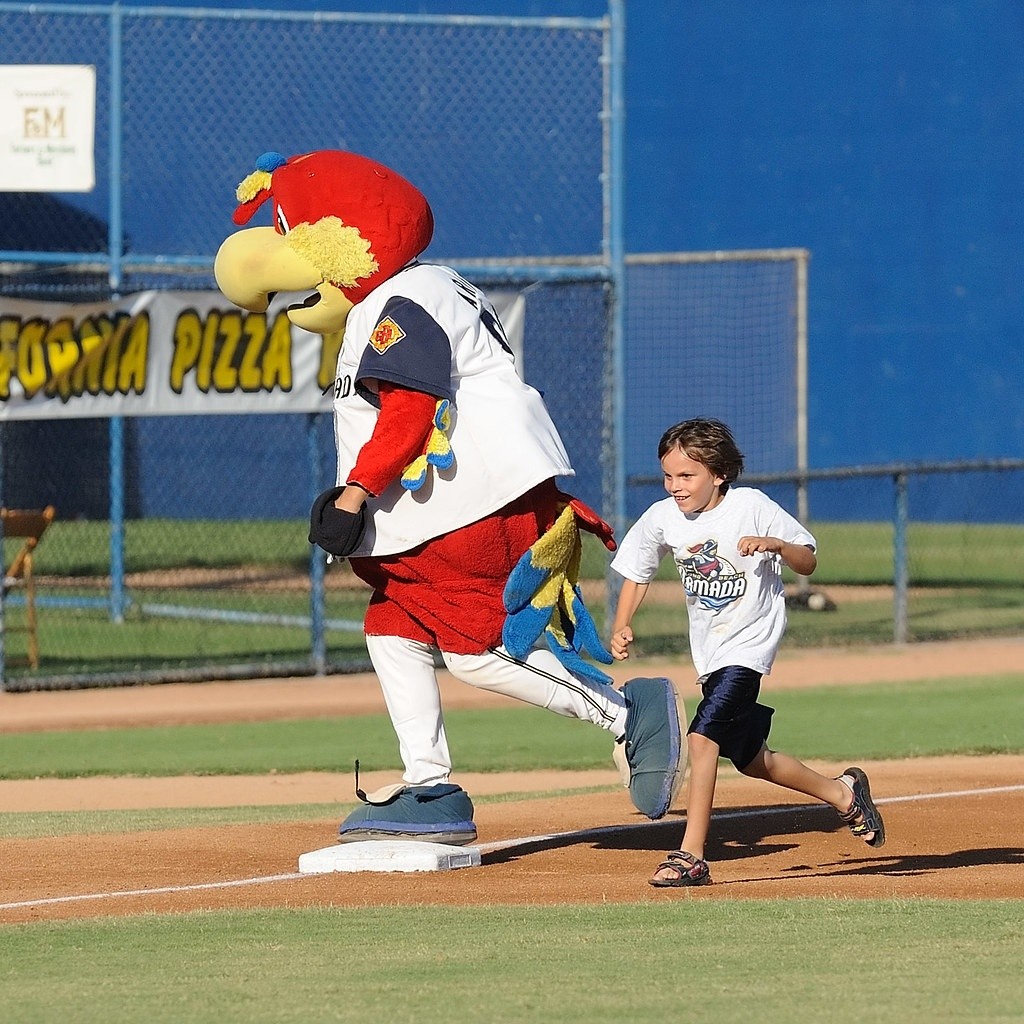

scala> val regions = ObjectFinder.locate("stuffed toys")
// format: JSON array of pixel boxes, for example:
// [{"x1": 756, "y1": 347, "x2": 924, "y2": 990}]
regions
[{"x1": 214, "y1": 149, "x2": 688, "y2": 844}]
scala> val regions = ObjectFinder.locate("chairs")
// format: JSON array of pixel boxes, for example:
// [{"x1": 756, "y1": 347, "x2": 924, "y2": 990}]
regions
[{"x1": 0, "y1": 505, "x2": 55, "y2": 669}]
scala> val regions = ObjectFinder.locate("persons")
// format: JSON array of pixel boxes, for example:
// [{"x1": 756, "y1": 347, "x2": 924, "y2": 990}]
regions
[{"x1": 610, "y1": 416, "x2": 887, "y2": 889}]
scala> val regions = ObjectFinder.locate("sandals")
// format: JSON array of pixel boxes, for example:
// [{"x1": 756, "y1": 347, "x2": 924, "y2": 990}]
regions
[
  {"x1": 649, "y1": 851, "x2": 710, "y2": 885},
  {"x1": 834, "y1": 767, "x2": 885, "y2": 847}
]
